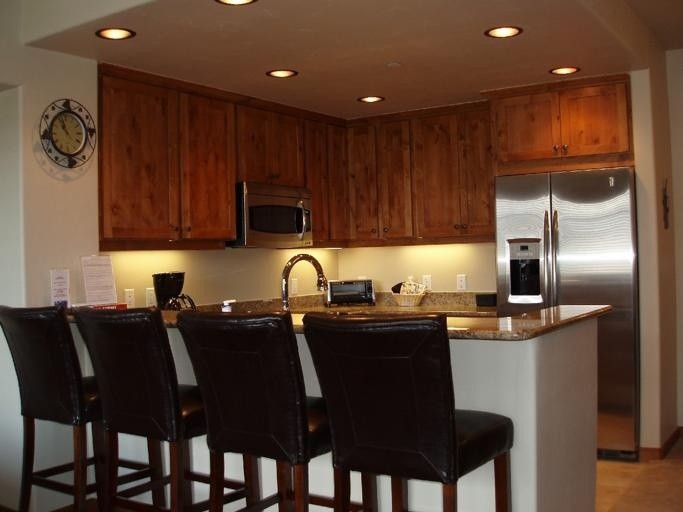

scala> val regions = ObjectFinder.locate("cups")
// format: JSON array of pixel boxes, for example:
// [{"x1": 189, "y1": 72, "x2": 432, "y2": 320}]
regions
[{"x1": 54, "y1": 300, "x2": 66, "y2": 314}]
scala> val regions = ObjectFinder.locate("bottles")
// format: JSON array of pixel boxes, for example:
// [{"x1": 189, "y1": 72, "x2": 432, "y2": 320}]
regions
[{"x1": 220, "y1": 301, "x2": 231, "y2": 312}]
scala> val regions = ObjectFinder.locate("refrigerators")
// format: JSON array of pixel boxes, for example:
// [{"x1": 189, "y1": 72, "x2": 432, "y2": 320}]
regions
[{"x1": 491, "y1": 167, "x2": 639, "y2": 461}]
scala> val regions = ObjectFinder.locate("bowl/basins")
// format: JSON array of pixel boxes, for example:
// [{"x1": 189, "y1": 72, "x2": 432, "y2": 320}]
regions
[{"x1": 70, "y1": 304, "x2": 95, "y2": 320}]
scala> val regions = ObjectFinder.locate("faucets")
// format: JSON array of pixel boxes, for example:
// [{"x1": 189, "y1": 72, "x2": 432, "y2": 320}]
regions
[{"x1": 281, "y1": 253, "x2": 328, "y2": 310}]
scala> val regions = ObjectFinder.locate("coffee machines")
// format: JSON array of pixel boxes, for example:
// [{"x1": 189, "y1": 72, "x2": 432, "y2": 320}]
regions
[{"x1": 151, "y1": 270, "x2": 196, "y2": 311}]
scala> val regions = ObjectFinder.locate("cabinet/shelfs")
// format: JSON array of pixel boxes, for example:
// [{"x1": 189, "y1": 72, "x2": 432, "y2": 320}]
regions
[
  {"x1": 346, "y1": 112, "x2": 414, "y2": 248},
  {"x1": 235, "y1": 92, "x2": 304, "y2": 184},
  {"x1": 409, "y1": 97, "x2": 495, "y2": 246},
  {"x1": 475, "y1": 69, "x2": 636, "y2": 179},
  {"x1": 95, "y1": 62, "x2": 240, "y2": 253},
  {"x1": 304, "y1": 108, "x2": 350, "y2": 251}
]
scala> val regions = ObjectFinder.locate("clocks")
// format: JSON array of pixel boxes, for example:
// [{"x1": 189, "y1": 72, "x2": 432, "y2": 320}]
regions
[{"x1": 32, "y1": 95, "x2": 100, "y2": 172}]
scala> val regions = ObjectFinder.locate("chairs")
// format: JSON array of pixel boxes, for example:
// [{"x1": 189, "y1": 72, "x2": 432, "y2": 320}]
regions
[
  {"x1": 73, "y1": 299, "x2": 263, "y2": 512},
  {"x1": 0, "y1": 299, "x2": 171, "y2": 512},
  {"x1": 300, "y1": 306, "x2": 517, "y2": 511},
  {"x1": 171, "y1": 302, "x2": 374, "y2": 511}
]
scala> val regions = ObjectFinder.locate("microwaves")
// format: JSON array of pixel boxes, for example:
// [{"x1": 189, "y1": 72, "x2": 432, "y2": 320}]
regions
[
  {"x1": 323, "y1": 279, "x2": 377, "y2": 308},
  {"x1": 229, "y1": 179, "x2": 316, "y2": 249}
]
